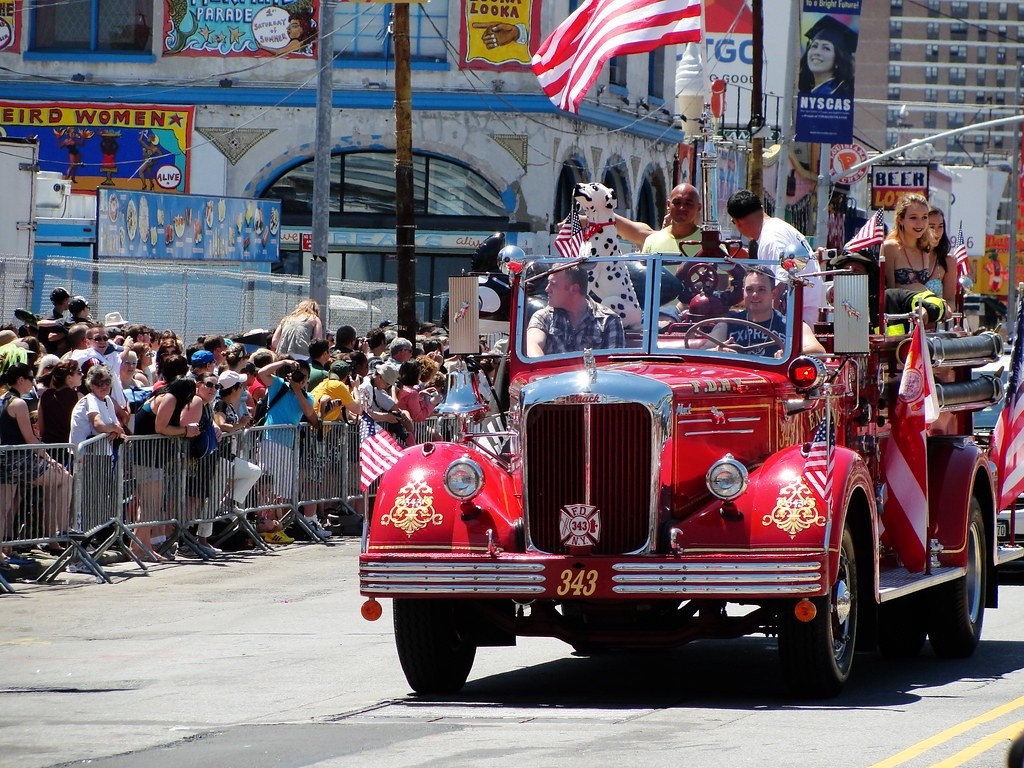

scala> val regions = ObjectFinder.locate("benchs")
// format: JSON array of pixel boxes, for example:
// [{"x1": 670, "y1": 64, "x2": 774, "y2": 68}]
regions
[{"x1": 624, "y1": 335, "x2": 708, "y2": 350}]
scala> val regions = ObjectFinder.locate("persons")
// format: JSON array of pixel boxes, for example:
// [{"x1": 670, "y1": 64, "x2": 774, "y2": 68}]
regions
[
  {"x1": 816, "y1": 193, "x2": 957, "y2": 335},
  {"x1": 0, "y1": 286, "x2": 500, "y2": 583},
  {"x1": 798, "y1": 15, "x2": 858, "y2": 97},
  {"x1": 698, "y1": 266, "x2": 825, "y2": 358},
  {"x1": 558, "y1": 182, "x2": 824, "y2": 332},
  {"x1": 527, "y1": 263, "x2": 624, "y2": 358}
]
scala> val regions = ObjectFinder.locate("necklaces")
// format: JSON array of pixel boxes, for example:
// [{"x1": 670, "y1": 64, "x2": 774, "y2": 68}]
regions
[{"x1": 899, "y1": 235, "x2": 925, "y2": 275}]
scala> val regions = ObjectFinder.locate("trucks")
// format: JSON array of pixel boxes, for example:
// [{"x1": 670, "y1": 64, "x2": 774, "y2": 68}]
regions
[{"x1": 358, "y1": 246, "x2": 1024, "y2": 695}]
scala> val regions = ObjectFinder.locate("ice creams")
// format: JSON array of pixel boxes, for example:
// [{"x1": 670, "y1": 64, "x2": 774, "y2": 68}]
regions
[{"x1": 676, "y1": 42, "x2": 705, "y2": 138}]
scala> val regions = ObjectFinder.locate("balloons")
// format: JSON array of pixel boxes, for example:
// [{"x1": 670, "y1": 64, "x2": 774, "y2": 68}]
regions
[{"x1": 441, "y1": 233, "x2": 548, "y2": 337}]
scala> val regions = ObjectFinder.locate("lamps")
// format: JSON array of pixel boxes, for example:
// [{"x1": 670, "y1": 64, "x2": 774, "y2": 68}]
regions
[
  {"x1": 659, "y1": 109, "x2": 671, "y2": 116},
  {"x1": 597, "y1": 85, "x2": 606, "y2": 97},
  {"x1": 70, "y1": 73, "x2": 86, "y2": 81},
  {"x1": 368, "y1": 82, "x2": 380, "y2": 89},
  {"x1": 673, "y1": 114, "x2": 687, "y2": 123},
  {"x1": 617, "y1": 97, "x2": 630, "y2": 106},
  {"x1": 636, "y1": 103, "x2": 650, "y2": 112},
  {"x1": 675, "y1": 118, "x2": 704, "y2": 125},
  {"x1": 218, "y1": 78, "x2": 233, "y2": 88}
]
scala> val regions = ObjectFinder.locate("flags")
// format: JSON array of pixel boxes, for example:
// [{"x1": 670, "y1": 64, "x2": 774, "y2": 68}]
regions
[
  {"x1": 951, "y1": 220, "x2": 970, "y2": 282},
  {"x1": 801, "y1": 407, "x2": 836, "y2": 518},
  {"x1": 359, "y1": 409, "x2": 405, "y2": 493},
  {"x1": 988, "y1": 298, "x2": 1024, "y2": 517},
  {"x1": 843, "y1": 207, "x2": 884, "y2": 253},
  {"x1": 554, "y1": 207, "x2": 584, "y2": 256},
  {"x1": 530, "y1": 0, "x2": 701, "y2": 115},
  {"x1": 878, "y1": 320, "x2": 938, "y2": 573}
]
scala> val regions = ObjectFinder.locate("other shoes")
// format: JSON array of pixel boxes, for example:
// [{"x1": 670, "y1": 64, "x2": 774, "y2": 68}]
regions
[
  {"x1": 0, "y1": 553, "x2": 22, "y2": 572},
  {"x1": 202, "y1": 544, "x2": 223, "y2": 554},
  {"x1": 218, "y1": 501, "x2": 245, "y2": 515},
  {"x1": 131, "y1": 541, "x2": 175, "y2": 563}
]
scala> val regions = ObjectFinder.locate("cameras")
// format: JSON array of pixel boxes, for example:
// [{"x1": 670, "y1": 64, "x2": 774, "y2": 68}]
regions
[
  {"x1": 430, "y1": 391, "x2": 439, "y2": 397},
  {"x1": 318, "y1": 397, "x2": 342, "y2": 414},
  {"x1": 345, "y1": 374, "x2": 363, "y2": 385},
  {"x1": 291, "y1": 369, "x2": 305, "y2": 383},
  {"x1": 388, "y1": 411, "x2": 409, "y2": 441},
  {"x1": 822, "y1": 248, "x2": 838, "y2": 261}
]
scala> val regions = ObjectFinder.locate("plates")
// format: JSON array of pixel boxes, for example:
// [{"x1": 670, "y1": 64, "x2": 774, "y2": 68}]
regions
[{"x1": 100, "y1": 188, "x2": 280, "y2": 243}]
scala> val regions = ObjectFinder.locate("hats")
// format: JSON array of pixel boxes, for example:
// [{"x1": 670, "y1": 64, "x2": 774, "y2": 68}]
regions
[
  {"x1": 330, "y1": 360, "x2": 350, "y2": 376},
  {"x1": 105, "y1": 311, "x2": 129, "y2": 327},
  {"x1": 49, "y1": 287, "x2": 74, "y2": 304},
  {"x1": 218, "y1": 370, "x2": 248, "y2": 390},
  {"x1": 375, "y1": 362, "x2": 399, "y2": 385},
  {"x1": 191, "y1": 350, "x2": 215, "y2": 367},
  {"x1": 379, "y1": 320, "x2": 398, "y2": 328},
  {"x1": 68, "y1": 296, "x2": 89, "y2": 315},
  {"x1": 15, "y1": 341, "x2": 38, "y2": 363}
]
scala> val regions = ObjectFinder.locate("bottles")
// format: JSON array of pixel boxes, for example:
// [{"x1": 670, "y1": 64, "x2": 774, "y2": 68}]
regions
[{"x1": 97, "y1": 195, "x2": 278, "y2": 254}]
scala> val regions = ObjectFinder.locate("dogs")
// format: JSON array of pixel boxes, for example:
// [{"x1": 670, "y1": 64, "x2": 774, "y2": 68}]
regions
[{"x1": 572, "y1": 182, "x2": 643, "y2": 331}]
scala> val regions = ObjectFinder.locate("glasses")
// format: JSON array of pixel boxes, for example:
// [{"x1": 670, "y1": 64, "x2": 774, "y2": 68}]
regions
[
  {"x1": 221, "y1": 345, "x2": 228, "y2": 348},
  {"x1": 93, "y1": 378, "x2": 111, "y2": 387},
  {"x1": 243, "y1": 353, "x2": 251, "y2": 360},
  {"x1": 88, "y1": 336, "x2": 108, "y2": 341},
  {"x1": 143, "y1": 331, "x2": 151, "y2": 336},
  {"x1": 731, "y1": 218, "x2": 736, "y2": 224},
  {"x1": 163, "y1": 349, "x2": 176, "y2": 356},
  {"x1": 200, "y1": 381, "x2": 220, "y2": 390},
  {"x1": 45, "y1": 365, "x2": 54, "y2": 369},
  {"x1": 86, "y1": 306, "x2": 91, "y2": 311},
  {"x1": 77, "y1": 369, "x2": 81, "y2": 375},
  {"x1": 404, "y1": 349, "x2": 413, "y2": 353},
  {"x1": 27, "y1": 376, "x2": 34, "y2": 383}
]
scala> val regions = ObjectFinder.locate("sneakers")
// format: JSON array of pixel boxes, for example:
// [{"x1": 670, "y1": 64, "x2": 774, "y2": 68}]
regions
[
  {"x1": 264, "y1": 530, "x2": 296, "y2": 545},
  {"x1": 67, "y1": 558, "x2": 102, "y2": 574},
  {"x1": 309, "y1": 520, "x2": 333, "y2": 538}
]
